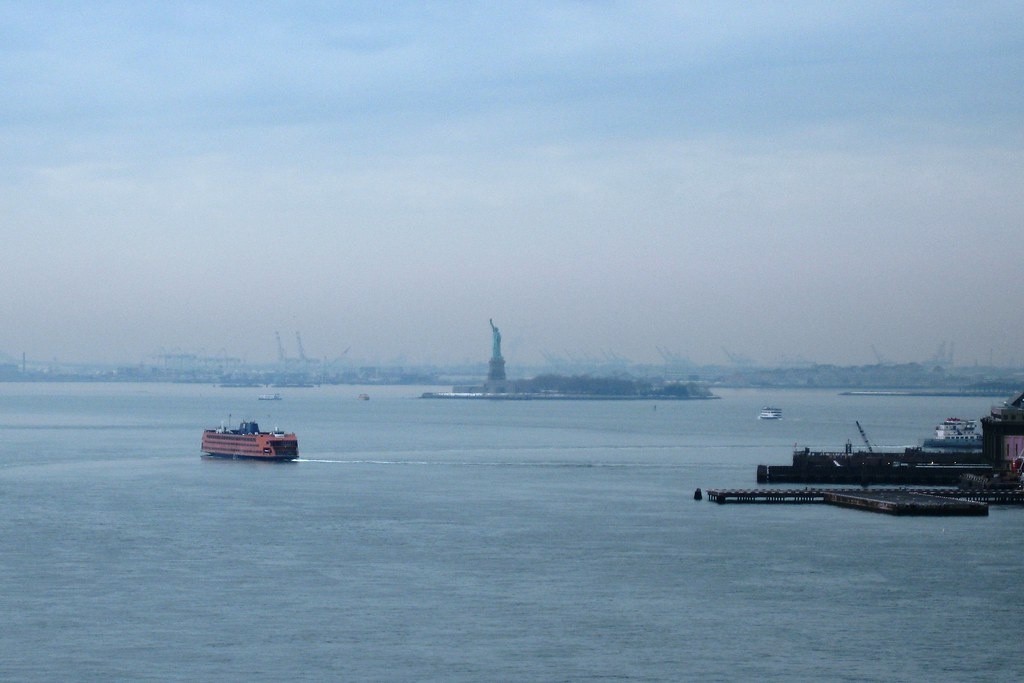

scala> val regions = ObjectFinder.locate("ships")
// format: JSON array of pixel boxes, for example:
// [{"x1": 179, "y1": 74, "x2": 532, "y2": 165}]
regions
[{"x1": 792, "y1": 447, "x2": 961, "y2": 466}]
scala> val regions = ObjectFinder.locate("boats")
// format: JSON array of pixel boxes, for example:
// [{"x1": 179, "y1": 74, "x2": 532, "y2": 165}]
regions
[
  {"x1": 201, "y1": 414, "x2": 299, "y2": 462},
  {"x1": 935, "y1": 416, "x2": 983, "y2": 440},
  {"x1": 258, "y1": 392, "x2": 283, "y2": 401},
  {"x1": 761, "y1": 406, "x2": 783, "y2": 419}
]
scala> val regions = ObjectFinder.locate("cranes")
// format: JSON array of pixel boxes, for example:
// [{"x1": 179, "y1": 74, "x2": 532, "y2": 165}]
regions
[
  {"x1": 855, "y1": 419, "x2": 873, "y2": 452},
  {"x1": 272, "y1": 329, "x2": 307, "y2": 365}
]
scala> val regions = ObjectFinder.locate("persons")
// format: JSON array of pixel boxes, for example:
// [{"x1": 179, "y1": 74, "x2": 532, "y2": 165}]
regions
[{"x1": 490, "y1": 318, "x2": 501, "y2": 358}]
